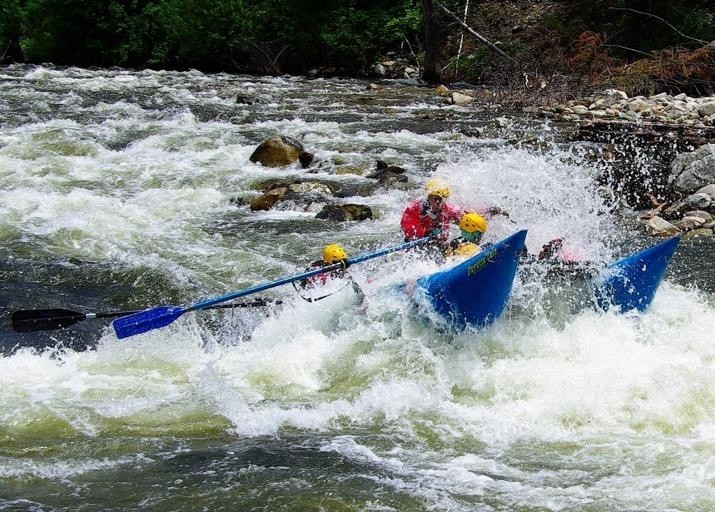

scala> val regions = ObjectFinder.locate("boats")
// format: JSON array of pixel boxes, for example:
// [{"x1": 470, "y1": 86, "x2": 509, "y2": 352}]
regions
[
  {"x1": 580, "y1": 236, "x2": 686, "y2": 307},
  {"x1": 288, "y1": 230, "x2": 534, "y2": 329}
]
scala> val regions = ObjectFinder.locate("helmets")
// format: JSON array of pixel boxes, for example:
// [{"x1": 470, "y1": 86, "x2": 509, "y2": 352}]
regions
[
  {"x1": 322, "y1": 244, "x2": 348, "y2": 265},
  {"x1": 424, "y1": 180, "x2": 450, "y2": 198},
  {"x1": 459, "y1": 213, "x2": 487, "y2": 233}
]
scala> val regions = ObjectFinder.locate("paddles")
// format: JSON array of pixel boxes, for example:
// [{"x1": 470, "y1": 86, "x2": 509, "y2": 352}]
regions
[
  {"x1": 12, "y1": 297, "x2": 282, "y2": 333},
  {"x1": 113, "y1": 236, "x2": 430, "y2": 339}
]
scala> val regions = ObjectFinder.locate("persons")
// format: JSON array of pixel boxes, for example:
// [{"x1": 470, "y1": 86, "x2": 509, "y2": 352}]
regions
[
  {"x1": 299, "y1": 245, "x2": 349, "y2": 290},
  {"x1": 400, "y1": 179, "x2": 505, "y2": 268}
]
